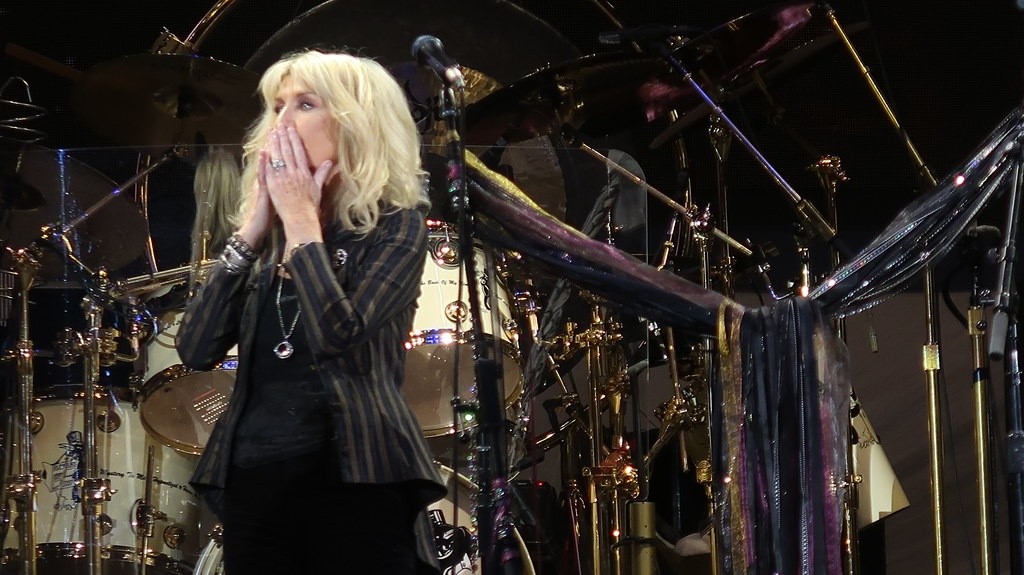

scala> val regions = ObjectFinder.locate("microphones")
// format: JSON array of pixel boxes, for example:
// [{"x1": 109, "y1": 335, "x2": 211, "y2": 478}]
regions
[
  {"x1": 411, "y1": 36, "x2": 467, "y2": 91},
  {"x1": 600, "y1": 25, "x2": 698, "y2": 45}
]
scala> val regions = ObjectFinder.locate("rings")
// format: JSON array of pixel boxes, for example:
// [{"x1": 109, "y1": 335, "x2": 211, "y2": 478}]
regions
[{"x1": 270, "y1": 158, "x2": 286, "y2": 168}]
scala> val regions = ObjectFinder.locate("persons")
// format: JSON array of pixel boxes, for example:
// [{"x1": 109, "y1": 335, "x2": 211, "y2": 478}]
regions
[{"x1": 174, "y1": 45, "x2": 444, "y2": 574}]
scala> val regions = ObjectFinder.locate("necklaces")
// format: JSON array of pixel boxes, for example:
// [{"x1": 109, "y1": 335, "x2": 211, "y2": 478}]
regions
[{"x1": 273, "y1": 245, "x2": 301, "y2": 361}]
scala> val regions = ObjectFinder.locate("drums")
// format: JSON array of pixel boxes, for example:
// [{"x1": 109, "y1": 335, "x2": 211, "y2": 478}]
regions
[
  {"x1": 191, "y1": 460, "x2": 538, "y2": 575},
  {"x1": 0, "y1": 382, "x2": 199, "y2": 575},
  {"x1": 402, "y1": 217, "x2": 523, "y2": 438},
  {"x1": 138, "y1": 299, "x2": 242, "y2": 455}
]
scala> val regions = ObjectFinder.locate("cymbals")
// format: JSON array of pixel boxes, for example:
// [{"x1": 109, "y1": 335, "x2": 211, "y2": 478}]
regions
[
  {"x1": 0, "y1": 135, "x2": 149, "y2": 279},
  {"x1": 454, "y1": 48, "x2": 677, "y2": 148},
  {"x1": 671, "y1": 1, "x2": 839, "y2": 66},
  {"x1": 79, "y1": 52, "x2": 260, "y2": 159}
]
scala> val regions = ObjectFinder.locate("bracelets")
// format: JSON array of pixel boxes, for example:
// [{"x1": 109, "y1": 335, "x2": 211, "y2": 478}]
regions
[{"x1": 220, "y1": 236, "x2": 258, "y2": 273}]
